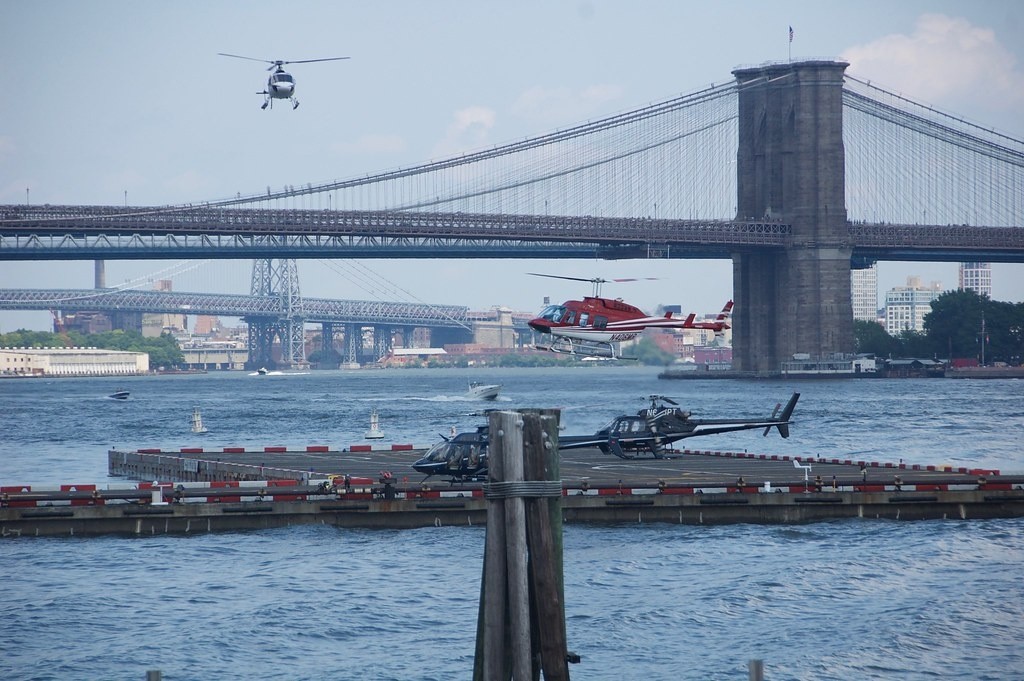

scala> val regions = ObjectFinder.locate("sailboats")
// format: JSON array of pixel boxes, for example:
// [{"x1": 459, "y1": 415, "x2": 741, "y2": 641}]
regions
[
  {"x1": 364, "y1": 408, "x2": 385, "y2": 438},
  {"x1": 190, "y1": 404, "x2": 209, "y2": 433}
]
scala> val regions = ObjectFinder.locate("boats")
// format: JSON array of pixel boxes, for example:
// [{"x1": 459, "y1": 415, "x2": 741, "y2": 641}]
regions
[
  {"x1": 464, "y1": 380, "x2": 503, "y2": 399},
  {"x1": 256, "y1": 368, "x2": 268, "y2": 375},
  {"x1": 109, "y1": 388, "x2": 129, "y2": 399}
]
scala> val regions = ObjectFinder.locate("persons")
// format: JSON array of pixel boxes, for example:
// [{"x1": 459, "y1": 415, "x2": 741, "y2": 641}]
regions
[{"x1": 344, "y1": 473, "x2": 352, "y2": 489}]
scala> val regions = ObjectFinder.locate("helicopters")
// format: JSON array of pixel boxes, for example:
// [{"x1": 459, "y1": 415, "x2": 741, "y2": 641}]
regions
[
  {"x1": 218, "y1": 48, "x2": 352, "y2": 110},
  {"x1": 410, "y1": 408, "x2": 669, "y2": 486},
  {"x1": 593, "y1": 392, "x2": 802, "y2": 460},
  {"x1": 524, "y1": 270, "x2": 734, "y2": 363}
]
